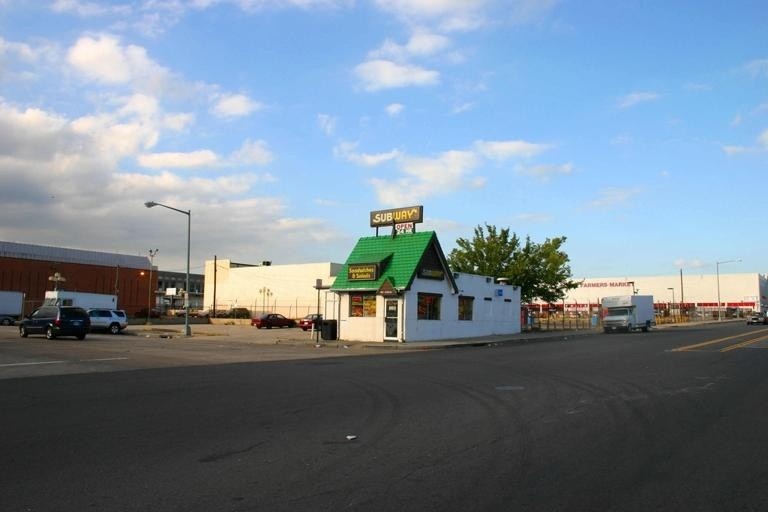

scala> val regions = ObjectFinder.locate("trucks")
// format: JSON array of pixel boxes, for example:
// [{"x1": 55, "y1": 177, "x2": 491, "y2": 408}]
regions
[
  {"x1": 599, "y1": 295, "x2": 655, "y2": 333},
  {"x1": 39, "y1": 290, "x2": 118, "y2": 312},
  {"x1": 0, "y1": 290, "x2": 25, "y2": 326}
]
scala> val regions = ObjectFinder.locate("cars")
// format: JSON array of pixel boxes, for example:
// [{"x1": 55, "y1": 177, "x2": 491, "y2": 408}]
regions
[
  {"x1": 176, "y1": 308, "x2": 249, "y2": 318},
  {"x1": 564, "y1": 311, "x2": 579, "y2": 318},
  {"x1": 732, "y1": 311, "x2": 742, "y2": 318},
  {"x1": 134, "y1": 306, "x2": 161, "y2": 320},
  {"x1": 297, "y1": 314, "x2": 322, "y2": 331},
  {"x1": 250, "y1": 313, "x2": 296, "y2": 329},
  {"x1": 577, "y1": 311, "x2": 588, "y2": 318},
  {"x1": 745, "y1": 309, "x2": 767, "y2": 325}
]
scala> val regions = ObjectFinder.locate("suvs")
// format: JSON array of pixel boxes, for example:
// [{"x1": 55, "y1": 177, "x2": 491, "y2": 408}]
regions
[
  {"x1": 19, "y1": 305, "x2": 90, "y2": 340},
  {"x1": 84, "y1": 308, "x2": 127, "y2": 334}
]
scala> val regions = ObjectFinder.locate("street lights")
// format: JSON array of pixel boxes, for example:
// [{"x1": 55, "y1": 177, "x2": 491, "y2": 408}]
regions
[
  {"x1": 48, "y1": 272, "x2": 67, "y2": 290},
  {"x1": 628, "y1": 281, "x2": 639, "y2": 294},
  {"x1": 667, "y1": 288, "x2": 675, "y2": 323},
  {"x1": 716, "y1": 259, "x2": 741, "y2": 322},
  {"x1": 147, "y1": 248, "x2": 159, "y2": 321},
  {"x1": 143, "y1": 201, "x2": 192, "y2": 337}
]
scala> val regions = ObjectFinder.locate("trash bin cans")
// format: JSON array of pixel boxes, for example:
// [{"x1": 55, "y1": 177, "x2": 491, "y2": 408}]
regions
[
  {"x1": 321, "y1": 320, "x2": 337, "y2": 340},
  {"x1": 593, "y1": 312, "x2": 600, "y2": 327}
]
